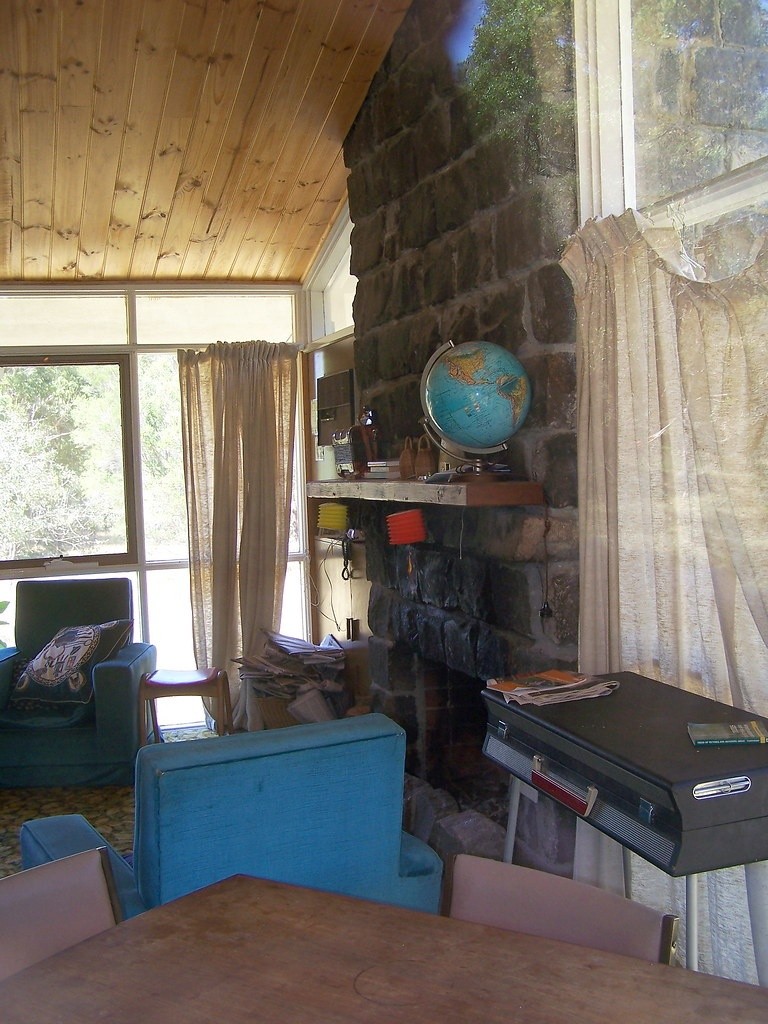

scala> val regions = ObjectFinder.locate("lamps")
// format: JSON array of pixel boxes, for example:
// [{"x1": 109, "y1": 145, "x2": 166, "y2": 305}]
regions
[
  {"x1": 316, "y1": 503, "x2": 365, "y2": 542},
  {"x1": 386, "y1": 508, "x2": 455, "y2": 554}
]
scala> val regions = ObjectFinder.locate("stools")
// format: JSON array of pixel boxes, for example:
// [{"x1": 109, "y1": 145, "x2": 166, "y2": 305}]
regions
[{"x1": 140, "y1": 669, "x2": 234, "y2": 748}]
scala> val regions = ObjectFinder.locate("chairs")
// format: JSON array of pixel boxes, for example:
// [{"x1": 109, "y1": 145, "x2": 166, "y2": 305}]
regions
[
  {"x1": 0, "y1": 845, "x2": 122, "y2": 982},
  {"x1": 448, "y1": 854, "x2": 680, "y2": 966}
]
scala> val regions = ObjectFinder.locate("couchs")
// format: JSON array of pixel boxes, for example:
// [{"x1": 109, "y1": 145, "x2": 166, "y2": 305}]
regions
[
  {"x1": 21, "y1": 714, "x2": 444, "y2": 933},
  {"x1": 0, "y1": 579, "x2": 165, "y2": 784}
]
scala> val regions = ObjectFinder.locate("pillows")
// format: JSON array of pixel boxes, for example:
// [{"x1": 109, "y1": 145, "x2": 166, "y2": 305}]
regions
[{"x1": 8, "y1": 618, "x2": 137, "y2": 706}]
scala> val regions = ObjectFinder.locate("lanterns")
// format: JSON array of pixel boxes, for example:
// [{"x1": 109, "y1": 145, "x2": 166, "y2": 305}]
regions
[
  {"x1": 317, "y1": 501, "x2": 350, "y2": 532},
  {"x1": 386, "y1": 508, "x2": 428, "y2": 546}
]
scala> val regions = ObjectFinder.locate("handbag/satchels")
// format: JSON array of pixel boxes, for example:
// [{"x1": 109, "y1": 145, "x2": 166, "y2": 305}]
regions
[
  {"x1": 415, "y1": 433, "x2": 436, "y2": 477},
  {"x1": 399, "y1": 436, "x2": 416, "y2": 480}
]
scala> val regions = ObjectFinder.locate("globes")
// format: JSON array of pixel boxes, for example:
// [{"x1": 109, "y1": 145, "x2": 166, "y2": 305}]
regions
[{"x1": 421, "y1": 340, "x2": 532, "y2": 482}]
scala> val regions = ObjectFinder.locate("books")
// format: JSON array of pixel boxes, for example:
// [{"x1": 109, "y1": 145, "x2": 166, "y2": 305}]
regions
[
  {"x1": 486, "y1": 668, "x2": 586, "y2": 697},
  {"x1": 362, "y1": 460, "x2": 402, "y2": 478},
  {"x1": 687, "y1": 721, "x2": 768, "y2": 748}
]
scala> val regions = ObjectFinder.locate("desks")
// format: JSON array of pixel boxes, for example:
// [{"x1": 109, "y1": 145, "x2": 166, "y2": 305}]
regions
[{"x1": 0, "y1": 874, "x2": 768, "y2": 1024}]
